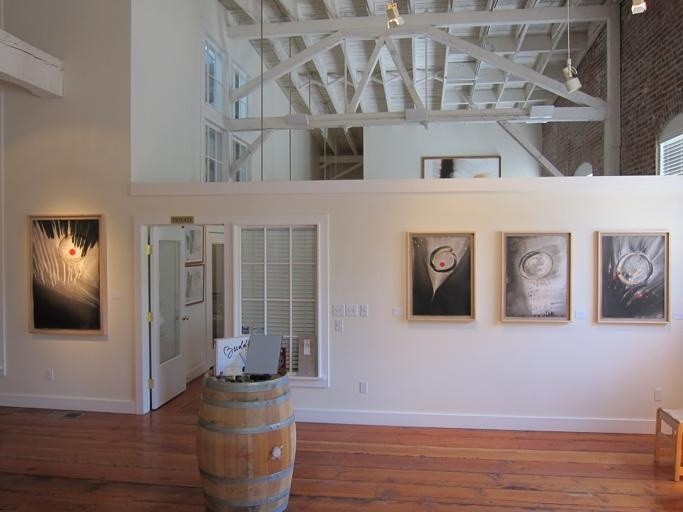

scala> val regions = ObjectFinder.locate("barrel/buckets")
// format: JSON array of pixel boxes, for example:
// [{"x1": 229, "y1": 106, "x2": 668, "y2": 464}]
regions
[{"x1": 196, "y1": 369, "x2": 297, "y2": 512}]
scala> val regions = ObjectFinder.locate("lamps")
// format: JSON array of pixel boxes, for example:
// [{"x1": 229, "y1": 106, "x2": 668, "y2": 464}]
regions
[{"x1": 561, "y1": 1, "x2": 581, "y2": 94}]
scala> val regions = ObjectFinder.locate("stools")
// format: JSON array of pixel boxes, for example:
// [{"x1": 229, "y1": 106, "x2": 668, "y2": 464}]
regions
[{"x1": 655, "y1": 407, "x2": 683, "y2": 482}]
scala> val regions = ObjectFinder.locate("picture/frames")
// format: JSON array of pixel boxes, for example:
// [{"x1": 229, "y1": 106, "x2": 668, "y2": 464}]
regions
[
  {"x1": 24, "y1": 212, "x2": 107, "y2": 337},
  {"x1": 421, "y1": 156, "x2": 501, "y2": 179},
  {"x1": 184, "y1": 225, "x2": 206, "y2": 306},
  {"x1": 214, "y1": 335, "x2": 250, "y2": 378},
  {"x1": 405, "y1": 229, "x2": 670, "y2": 323}
]
